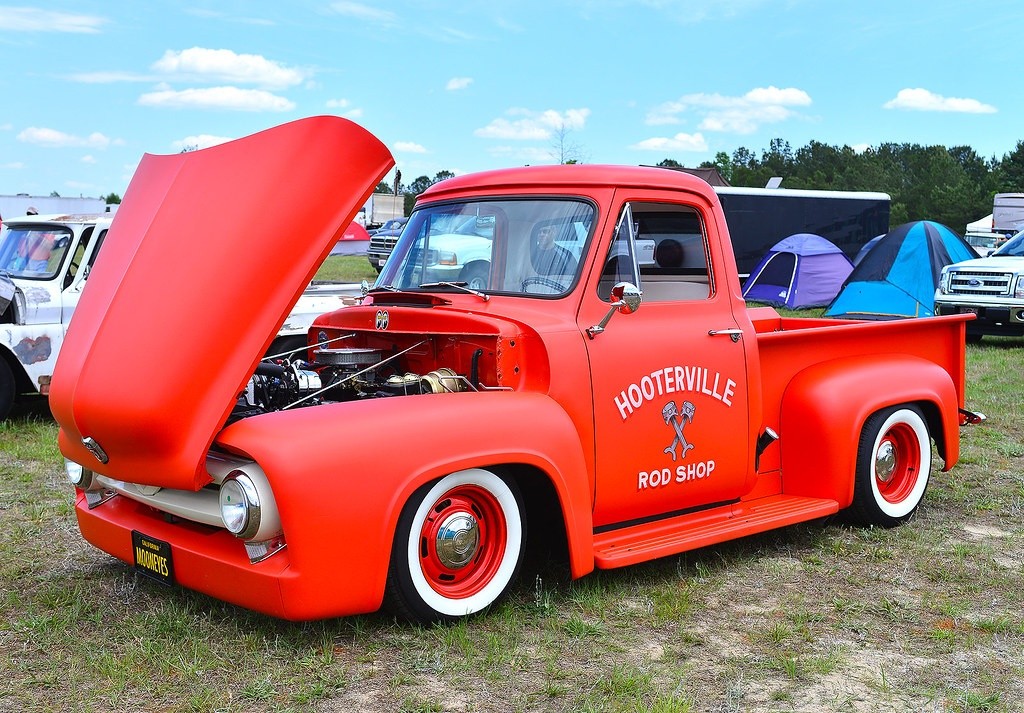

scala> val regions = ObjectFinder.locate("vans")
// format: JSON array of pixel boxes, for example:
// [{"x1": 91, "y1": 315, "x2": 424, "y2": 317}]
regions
[{"x1": 963, "y1": 233, "x2": 1006, "y2": 258}]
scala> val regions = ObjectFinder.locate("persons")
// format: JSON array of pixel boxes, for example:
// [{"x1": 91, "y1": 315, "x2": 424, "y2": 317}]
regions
[
  {"x1": 533, "y1": 222, "x2": 576, "y2": 274},
  {"x1": 997, "y1": 233, "x2": 1012, "y2": 249}
]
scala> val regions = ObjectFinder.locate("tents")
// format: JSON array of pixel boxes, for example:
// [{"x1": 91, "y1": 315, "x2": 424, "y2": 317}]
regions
[
  {"x1": 820, "y1": 220, "x2": 985, "y2": 321},
  {"x1": 741, "y1": 233, "x2": 857, "y2": 312}
]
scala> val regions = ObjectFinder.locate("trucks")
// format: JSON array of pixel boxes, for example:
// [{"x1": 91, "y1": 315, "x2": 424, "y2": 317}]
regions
[{"x1": 991, "y1": 193, "x2": 1024, "y2": 241}]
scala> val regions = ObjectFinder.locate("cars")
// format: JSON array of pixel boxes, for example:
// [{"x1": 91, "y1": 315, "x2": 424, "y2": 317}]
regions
[
  {"x1": 367, "y1": 218, "x2": 411, "y2": 237},
  {"x1": 933, "y1": 228, "x2": 1024, "y2": 345}
]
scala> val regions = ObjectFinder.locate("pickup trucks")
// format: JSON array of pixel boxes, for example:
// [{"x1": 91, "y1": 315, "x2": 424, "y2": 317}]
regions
[
  {"x1": 368, "y1": 211, "x2": 476, "y2": 274},
  {"x1": 49, "y1": 114, "x2": 987, "y2": 626},
  {"x1": 402, "y1": 213, "x2": 657, "y2": 291},
  {"x1": 0, "y1": 212, "x2": 375, "y2": 416}
]
